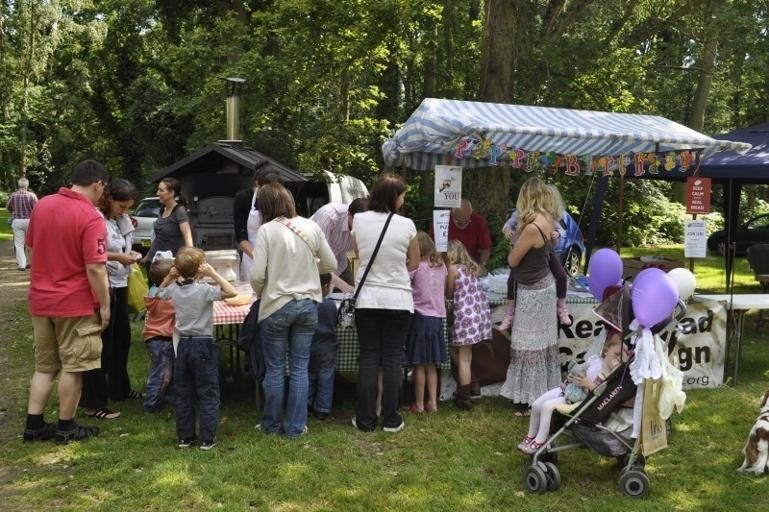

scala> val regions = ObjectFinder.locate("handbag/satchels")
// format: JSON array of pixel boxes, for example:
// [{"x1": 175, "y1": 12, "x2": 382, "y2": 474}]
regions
[
  {"x1": 640, "y1": 372, "x2": 668, "y2": 457},
  {"x1": 336, "y1": 292, "x2": 356, "y2": 332}
]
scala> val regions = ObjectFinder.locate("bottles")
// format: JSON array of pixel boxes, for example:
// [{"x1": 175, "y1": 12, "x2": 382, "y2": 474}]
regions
[{"x1": 223, "y1": 261, "x2": 237, "y2": 292}]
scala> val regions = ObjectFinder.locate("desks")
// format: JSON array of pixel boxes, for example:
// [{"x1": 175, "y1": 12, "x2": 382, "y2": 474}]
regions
[
  {"x1": 213, "y1": 279, "x2": 729, "y2": 420},
  {"x1": 693, "y1": 294, "x2": 769, "y2": 386}
]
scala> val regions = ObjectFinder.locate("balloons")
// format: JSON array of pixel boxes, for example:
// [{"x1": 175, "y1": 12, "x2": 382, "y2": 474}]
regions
[
  {"x1": 667, "y1": 267, "x2": 696, "y2": 301},
  {"x1": 588, "y1": 248, "x2": 623, "y2": 301},
  {"x1": 588, "y1": 277, "x2": 623, "y2": 305},
  {"x1": 632, "y1": 271, "x2": 679, "y2": 328},
  {"x1": 632, "y1": 267, "x2": 666, "y2": 284}
]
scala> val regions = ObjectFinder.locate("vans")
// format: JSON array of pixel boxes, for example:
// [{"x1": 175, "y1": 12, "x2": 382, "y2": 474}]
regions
[{"x1": 130, "y1": 196, "x2": 183, "y2": 250}]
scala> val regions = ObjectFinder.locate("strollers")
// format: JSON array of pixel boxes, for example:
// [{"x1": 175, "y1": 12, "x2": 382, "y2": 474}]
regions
[{"x1": 525, "y1": 274, "x2": 689, "y2": 496}]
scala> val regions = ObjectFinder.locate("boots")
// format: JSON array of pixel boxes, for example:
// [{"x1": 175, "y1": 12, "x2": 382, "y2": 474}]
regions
[
  {"x1": 455, "y1": 384, "x2": 472, "y2": 410},
  {"x1": 471, "y1": 378, "x2": 481, "y2": 396}
]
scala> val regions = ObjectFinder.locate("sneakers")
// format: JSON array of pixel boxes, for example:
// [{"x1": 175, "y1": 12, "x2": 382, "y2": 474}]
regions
[
  {"x1": 23, "y1": 422, "x2": 55, "y2": 442},
  {"x1": 53, "y1": 424, "x2": 99, "y2": 446},
  {"x1": 350, "y1": 416, "x2": 406, "y2": 434},
  {"x1": 200, "y1": 441, "x2": 216, "y2": 450},
  {"x1": 179, "y1": 438, "x2": 195, "y2": 448},
  {"x1": 254, "y1": 404, "x2": 328, "y2": 435},
  {"x1": 557, "y1": 303, "x2": 572, "y2": 326},
  {"x1": 497, "y1": 312, "x2": 512, "y2": 331}
]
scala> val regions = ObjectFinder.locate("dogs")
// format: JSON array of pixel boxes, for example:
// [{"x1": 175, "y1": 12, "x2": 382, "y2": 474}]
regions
[{"x1": 735, "y1": 387, "x2": 769, "y2": 476}]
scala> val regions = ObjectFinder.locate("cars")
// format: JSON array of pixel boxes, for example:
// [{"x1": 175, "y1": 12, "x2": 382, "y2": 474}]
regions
[
  {"x1": 502, "y1": 207, "x2": 587, "y2": 279},
  {"x1": 708, "y1": 213, "x2": 768, "y2": 258}
]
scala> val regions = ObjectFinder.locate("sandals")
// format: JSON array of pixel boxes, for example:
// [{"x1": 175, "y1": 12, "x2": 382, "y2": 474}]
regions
[
  {"x1": 513, "y1": 404, "x2": 533, "y2": 417},
  {"x1": 523, "y1": 438, "x2": 547, "y2": 454},
  {"x1": 518, "y1": 435, "x2": 535, "y2": 450},
  {"x1": 83, "y1": 407, "x2": 120, "y2": 420},
  {"x1": 407, "y1": 403, "x2": 437, "y2": 414},
  {"x1": 120, "y1": 390, "x2": 147, "y2": 399}
]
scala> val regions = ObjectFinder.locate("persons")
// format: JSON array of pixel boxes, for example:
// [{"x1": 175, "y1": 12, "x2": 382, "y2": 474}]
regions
[
  {"x1": 498, "y1": 185, "x2": 572, "y2": 331},
  {"x1": 6, "y1": 177, "x2": 39, "y2": 271},
  {"x1": 498, "y1": 177, "x2": 562, "y2": 417},
  {"x1": 517, "y1": 333, "x2": 628, "y2": 455},
  {"x1": 23, "y1": 158, "x2": 112, "y2": 444}
]
